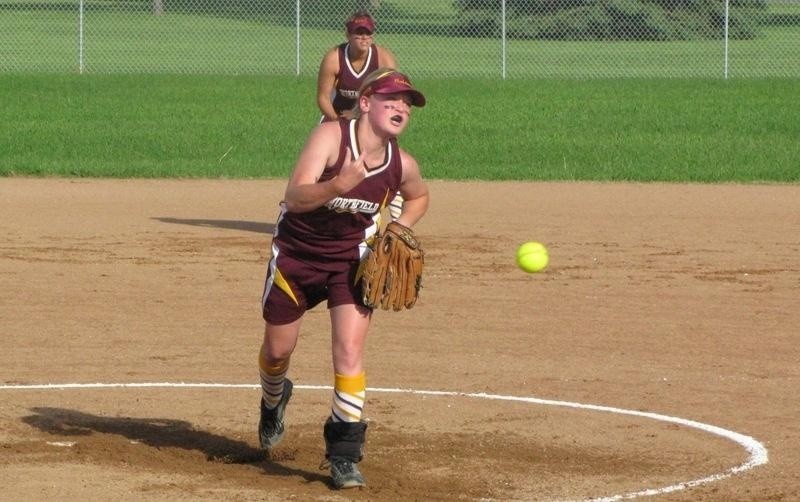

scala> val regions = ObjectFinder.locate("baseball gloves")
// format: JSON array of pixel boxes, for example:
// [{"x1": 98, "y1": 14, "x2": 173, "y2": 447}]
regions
[{"x1": 353, "y1": 222, "x2": 423, "y2": 311}]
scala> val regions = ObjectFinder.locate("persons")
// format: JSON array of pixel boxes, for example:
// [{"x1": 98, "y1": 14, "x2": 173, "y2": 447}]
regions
[
  {"x1": 315, "y1": 9, "x2": 405, "y2": 223},
  {"x1": 256, "y1": 65, "x2": 431, "y2": 490}
]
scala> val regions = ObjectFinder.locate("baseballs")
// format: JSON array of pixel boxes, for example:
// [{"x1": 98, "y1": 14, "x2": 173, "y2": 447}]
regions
[{"x1": 517, "y1": 242, "x2": 549, "y2": 272}]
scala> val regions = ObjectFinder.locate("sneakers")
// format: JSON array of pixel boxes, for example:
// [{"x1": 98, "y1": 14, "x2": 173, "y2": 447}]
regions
[
  {"x1": 256, "y1": 378, "x2": 293, "y2": 449},
  {"x1": 319, "y1": 456, "x2": 367, "y2": 492}
]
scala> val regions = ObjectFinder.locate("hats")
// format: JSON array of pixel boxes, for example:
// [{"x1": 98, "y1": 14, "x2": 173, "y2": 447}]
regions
[
  {"x1": 357, "y1": 70, "x2": 426, "y2": 110},
  {"x1": 346, "y1": 15, "x2": 375, "y2": 34}
]
